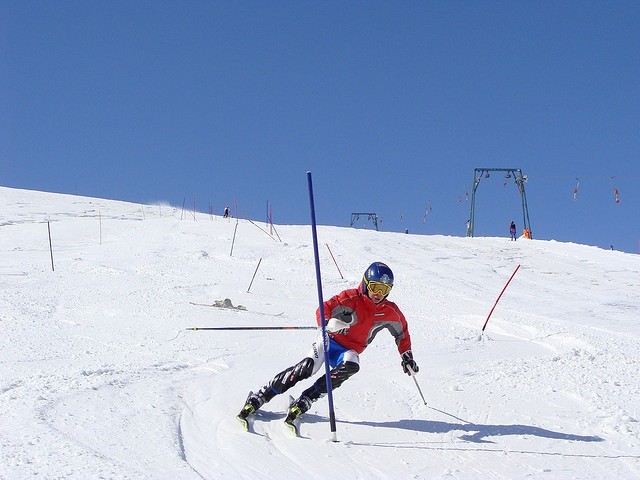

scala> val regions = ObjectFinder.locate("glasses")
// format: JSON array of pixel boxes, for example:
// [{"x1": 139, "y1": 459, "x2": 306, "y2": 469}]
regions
[{"x1": 363, "y1": 274, "x2": 391, "y2": 296}]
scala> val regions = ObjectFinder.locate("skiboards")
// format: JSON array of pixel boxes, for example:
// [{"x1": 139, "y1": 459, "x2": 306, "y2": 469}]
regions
[{"x1": 236, "y1": 391, "x2": 299, "y2": 437}]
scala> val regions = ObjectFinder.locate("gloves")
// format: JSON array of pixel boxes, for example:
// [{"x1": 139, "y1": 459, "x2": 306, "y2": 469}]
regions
[
  {"x1": 327, "y1": 317, "x2": 350, "y2": 335},
  {"x1": 401, "y1": 349, "x2": 420, "y2": 376}
]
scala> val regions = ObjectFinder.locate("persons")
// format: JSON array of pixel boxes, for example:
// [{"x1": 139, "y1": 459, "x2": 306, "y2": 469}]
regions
[
  {"x1": 244, "y1": 262, "x2": 419, "y2": 417},
  {"x1": 466, "y1": 220, "x2": 471, "y2": 237},
  {"x1": 223, "y1": 206, "x2": 230, "y2": 218},
  {"x1": 509, "y1": 220, "x2": 516, "y2": 241}
]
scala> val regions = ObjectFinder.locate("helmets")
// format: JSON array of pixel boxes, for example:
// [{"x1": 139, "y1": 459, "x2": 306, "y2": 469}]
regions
[{"x1": 361, "y1": 263, "x2": 394, "y2": 297}]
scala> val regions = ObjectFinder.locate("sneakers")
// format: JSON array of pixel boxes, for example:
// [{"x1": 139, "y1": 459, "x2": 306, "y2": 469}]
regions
[
  {"x1": 291, "y1": 395, "x2": 312, "y2": 418},
  {"x1": 246, "y1": 391, "x2": 268, "y2": 413}
]
